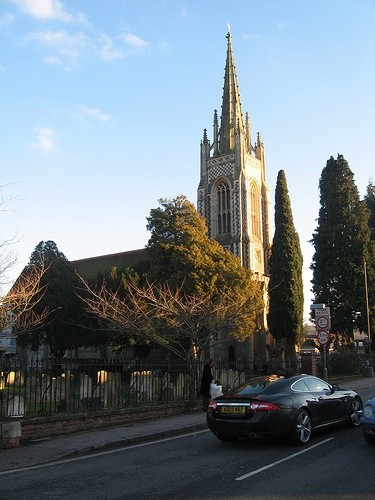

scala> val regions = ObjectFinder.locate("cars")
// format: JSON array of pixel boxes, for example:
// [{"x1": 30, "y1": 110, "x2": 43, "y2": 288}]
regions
[
  {"x1": 206, "y1": 372, "x2": 364, "y2": 448},
  {"x1": 300, "y1": 345, "x2": 321, "y2": 356},
  {"x1": 362, "y1": 395, "x2": 375, "y2": 444}
]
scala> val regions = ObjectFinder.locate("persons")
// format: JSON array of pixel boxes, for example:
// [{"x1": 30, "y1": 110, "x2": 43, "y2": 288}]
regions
[
  {"x1": 200, "y1": 358, "x2": 215, "y2": 410},
  {"x1": 208, "y1": 380, "x2": 224, "y2": 402}
]
